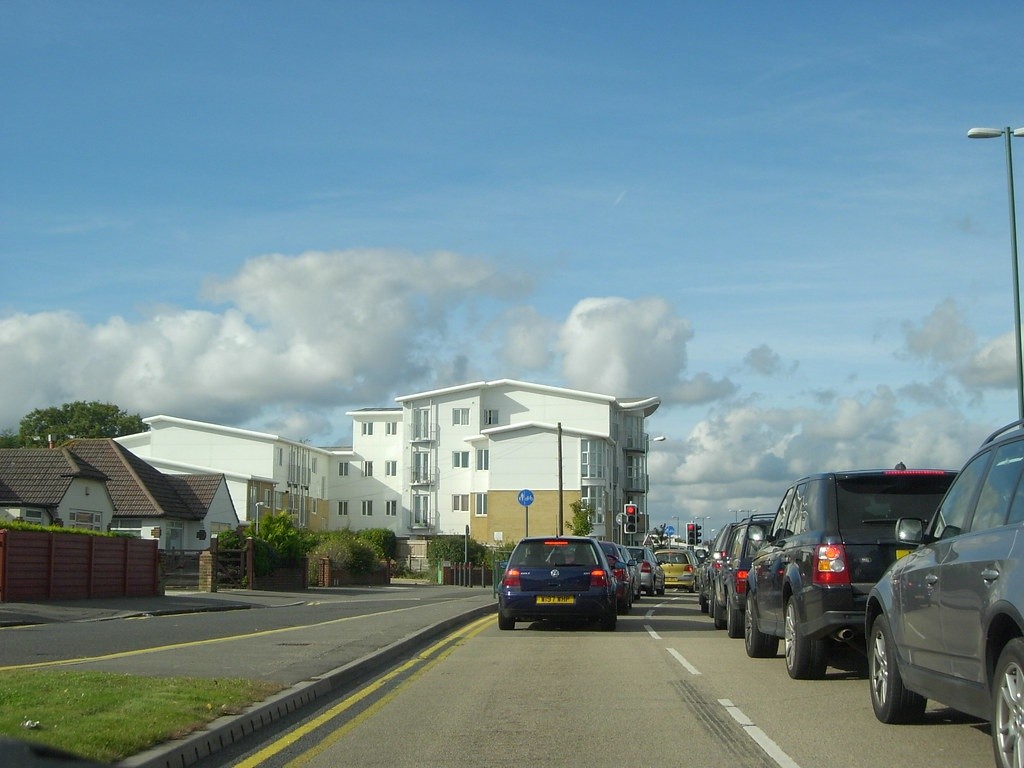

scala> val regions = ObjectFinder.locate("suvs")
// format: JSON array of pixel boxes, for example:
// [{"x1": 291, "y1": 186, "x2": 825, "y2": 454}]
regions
[
  {"x1": 865, "y1": 420, "x2": 1024, "y2": 768},
  {"x1": 714, "y1": 513, "x2": 777, "y2": 639}
]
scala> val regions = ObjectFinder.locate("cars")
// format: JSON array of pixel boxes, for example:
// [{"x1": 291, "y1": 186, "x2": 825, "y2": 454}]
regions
[
  {"x1": 608, "y1": 545, "x2": 641, "y2": 603},
  {"x1": 625, "y1": 547, "x2": 665, "y2": 596},
  {"x1": 695, "y1": 523, "x2": 739, "y2": 618},
  {"x1": 655, "y1": 549, "x2": 701, "y2": 593},
  {"x1": 665, "y1": 550, "x2": 702, "y2": 591},
  {"x1": 545, "y1": 541, "x2": 638, "y2": 615},
  {"x1": 497, "y1": 537, "x2": 617, "y2": 631},
  {"x1": 744, "y1": 468, "x2": 962, "y2": 680}
]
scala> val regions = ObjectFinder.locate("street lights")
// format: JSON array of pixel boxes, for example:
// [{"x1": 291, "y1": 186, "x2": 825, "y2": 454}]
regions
[
  {"x1": 695, "y1": 517, "x2": 711, "y2": 544},
  {"x1": 739, "y1": 509, "x2": 757, "y2": 517},
  {"x1": 709, "y1": 529, "x2": 715, "y2": 544},
  {"x1": 672, "y1": 516, "x2": 679, "y2": 549},
  {"x1": 644, "y1": 435, "x2": 666, "y2": 536},
  {"x1": 968, "y1": 127, "x2": 1024, "y2": 427},
  {"x1": 255, "y1": 502, "x2": 265, "y2": 536},
  {"x1": 729, "y1": 510, "x2": 744, "y2": 522}
]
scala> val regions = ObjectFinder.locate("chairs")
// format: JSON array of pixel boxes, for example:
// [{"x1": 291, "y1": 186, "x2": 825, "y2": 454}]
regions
[
  {"x1": 794, "y1": 496, "x2": 937, "y2": 528},
  {"x1": 1003, "y1": 493, "x2": 1024, "y2": 525},
  {"x1": 524, "y1": 552, "x2": 594, "y2": 566}
]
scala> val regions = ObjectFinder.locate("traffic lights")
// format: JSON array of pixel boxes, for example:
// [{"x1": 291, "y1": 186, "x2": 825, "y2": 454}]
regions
[
  {"x1": 695, "y1": 523, "x2": 702, "y2": 545},
  {"x1": 686, "y1": 524, "x2": 696, "y2": 546},
  {"x1": 624, "y1": 504, "x2": 637, "y2": 533}
]
